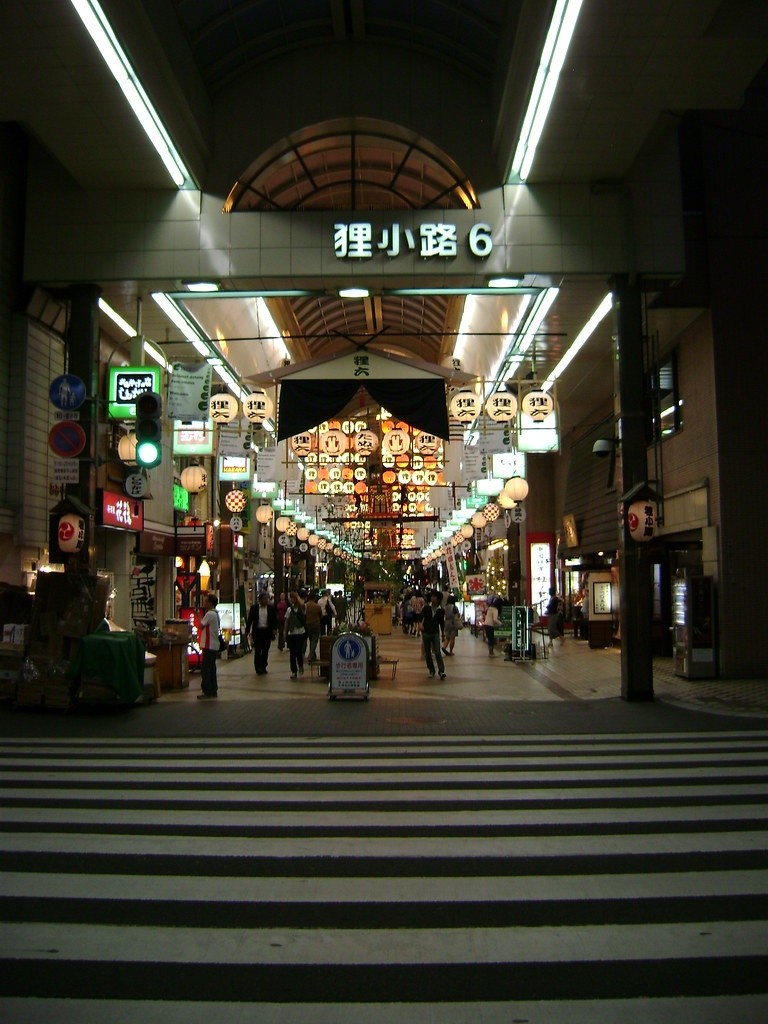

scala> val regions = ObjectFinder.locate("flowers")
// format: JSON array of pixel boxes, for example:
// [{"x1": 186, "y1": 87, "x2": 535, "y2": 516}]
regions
[{"x1": 327, "y1": 619, "x2": 372, "y2": 636}]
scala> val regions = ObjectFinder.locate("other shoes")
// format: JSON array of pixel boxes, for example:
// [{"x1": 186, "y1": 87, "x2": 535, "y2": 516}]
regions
[
  {"x1": 450, "y1": 652, "x2": 454, "y2": 655},
  {"x1": 441, "y1": 647, "x2": 449, "y2": 654},
  {"x1": 197, "y1": 694, "x2": 217, "y2": 699},
  {"x1": 429, "y1": 672, "x2": 434, "y2": 677},
  {"x1": 299, "y1": 667, "x2": 304, "y2": 674},
  {"x1": 290, "y1": 672, "x2": 297, "y2": 678},
  {"x1": 440, "y1": 672, "x2": 446, "y2": 679}
]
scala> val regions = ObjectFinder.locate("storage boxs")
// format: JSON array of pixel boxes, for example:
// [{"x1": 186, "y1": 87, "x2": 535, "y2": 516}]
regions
[{"x1": 2, "y1": 596, "x2": 87, "y2": 707}]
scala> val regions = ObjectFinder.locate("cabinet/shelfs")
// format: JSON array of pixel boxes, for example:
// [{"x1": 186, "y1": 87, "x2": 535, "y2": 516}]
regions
[
  {"x1": 0, "y1": 642, "x2": 24, "y2": 702},
  {"x1": 320, "y1": 633, "x2": 380, "y2": 678},
  {"x1": 16, "y1": 572, "x2": 110, "y2": 705}
]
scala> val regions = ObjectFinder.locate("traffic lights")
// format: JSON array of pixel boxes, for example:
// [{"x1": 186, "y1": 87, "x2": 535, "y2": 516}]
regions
[{"x1": 135, "y1": 391, "x2": 167, "y2": 470}]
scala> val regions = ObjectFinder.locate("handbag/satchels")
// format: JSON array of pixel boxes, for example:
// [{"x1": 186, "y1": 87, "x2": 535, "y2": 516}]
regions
[
  {"x1": 454, "y1": 607, "x2": 464, "y2": 630},
  {"x1": 216, "y1": 634, "x2": 227, "y2": 652},
  {"x1": 326, "y1": 599, "x2": 333, "y2": 614}
]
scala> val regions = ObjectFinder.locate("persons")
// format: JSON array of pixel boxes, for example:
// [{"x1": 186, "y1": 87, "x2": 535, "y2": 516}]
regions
[
  {"x1": 398, "y1": 586, "x2": 460, "y2": 678},
  {"x1": 581, "y1": 589, "x2": 589, "y2": 640},
  {"x1": 194, "y1": 594, "x2": 220, "y2": 698},
  {"x1": 546, "y1": 587, "x2": 566, "y2": 646},
  {"x1": 245, "y1": 589, "x2": 347, "y2": 678},
  {"x1": 485, "y1": 599, "x2": 503, "y2": 657}
]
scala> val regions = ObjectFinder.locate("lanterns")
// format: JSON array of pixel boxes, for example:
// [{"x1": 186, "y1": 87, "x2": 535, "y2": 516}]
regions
[
  {"x1": 629, "y1": 501, "x2": 657, "y2": 541},
  {"x1": 225, "y1": 488, "x2": 247, "y2": 514},
  {"x1": 487, "y1": 390, "x2": 517, "y2": 423},
  {"x1": 230, "y1": 516, "x2": 242, "y2": 531},
  {"x1": 292, "y1": 421, "x2": 447, "y2": 560},
  {"x1": 522, "y1": 390, "x2": 553, "y2": 422},
  {"x1": 422, "y1": 491, "x2": 525, "y2": 570},
  {"x1": 209, "y1": 392, "x2": 238, "y2": 426},
  {"x1": 244, "y1": 392, "x2": 273, "y2": 425},
  {"x1": 505, "y1": 476, "x2": 528, "y2": 504},
  {"x1": 255, "y1": 503, "x2": 361, "y2": 566},
  {"x1": 118, "y1": 435, "x2": 139, "y2": 466},
  {"x1": 181, "y1": 464, "x2": 208, "y2": 495},
  {"x1": 58, "y1": 512, "x2": 85, "y2": 552},
  {"x1": 451, "y1": 390, "x2": 481, "y2": 423}
]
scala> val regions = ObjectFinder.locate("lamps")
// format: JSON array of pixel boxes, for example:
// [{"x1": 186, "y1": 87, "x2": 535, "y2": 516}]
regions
[
  {"x1": 217, "y1": 602, "x2": 240, "y2": 645},
  {"x1": 196, "y1": 559, "x2": 211, "y2": 594}
]
